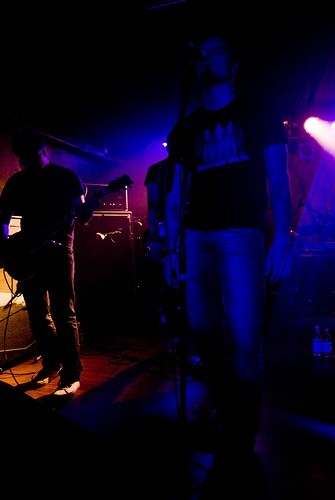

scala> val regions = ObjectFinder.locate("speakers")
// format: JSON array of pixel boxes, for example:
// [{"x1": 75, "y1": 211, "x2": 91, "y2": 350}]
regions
[{"x1": 73, "y1": 213, "x2": 138, "y2": 325}]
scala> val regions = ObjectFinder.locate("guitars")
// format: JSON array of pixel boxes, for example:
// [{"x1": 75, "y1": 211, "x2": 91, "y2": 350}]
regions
[{"x1": 2, "y1": 173, "x2": 134, "y2": 281}]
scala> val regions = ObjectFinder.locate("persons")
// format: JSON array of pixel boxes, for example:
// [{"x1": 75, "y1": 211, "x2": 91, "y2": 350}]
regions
[
  {"x1": 0, "y1": 131, "x2": 100, "y2": 402},
  {"x1": 165, "y1": 31, "x2": 292, "y2": 396},
  {"x1": 145, "y1": 133, "x2": 183, "y2": 299}
]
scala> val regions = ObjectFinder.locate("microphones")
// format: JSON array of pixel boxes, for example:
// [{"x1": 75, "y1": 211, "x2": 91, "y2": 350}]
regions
[{"x1": 190, "y1": 42, "x2": 212, "y2": 64}]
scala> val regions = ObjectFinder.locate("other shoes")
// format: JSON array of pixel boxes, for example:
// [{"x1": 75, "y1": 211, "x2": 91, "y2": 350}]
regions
[
  {"x1": 50, "y1": 380, "x2": 80, "y2": 400},
  {"x1": 32, "y1": 367, "x2": 62, "y2": 387}
]
